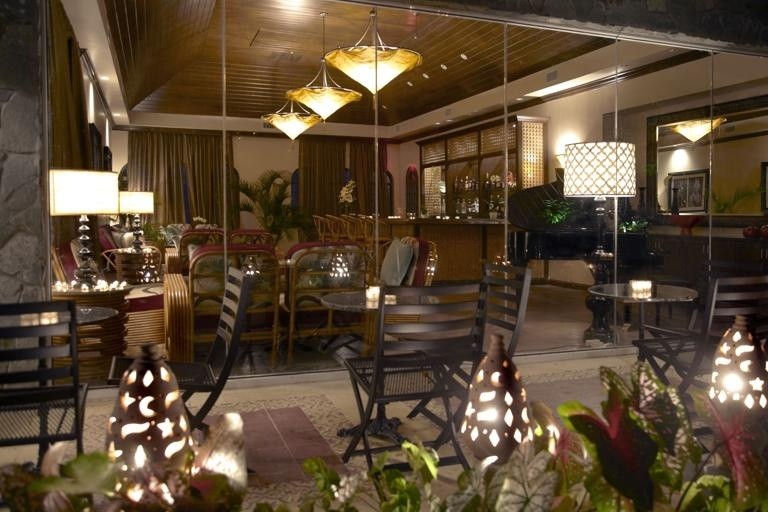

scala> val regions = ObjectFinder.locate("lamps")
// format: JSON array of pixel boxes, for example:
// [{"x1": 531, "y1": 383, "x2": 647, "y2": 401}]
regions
[
  {"x1": 562, "y1": 142, "x2": 636, "y2": 347},
  {"x1": 117, "y1": 190, "x2": 154, "y2": 250},
  {"x1": 49, "y1": 168, "x2": 120, "y2": 288},
  {"x1": 554, "y1": 132, "x2": 582, "y2": 167},
  {"x1": 260, "y1": 9, "x2": 423, "y2": 142},
  {"x1": 669, "y1": 118, "x2": 726, "y2": 143}
]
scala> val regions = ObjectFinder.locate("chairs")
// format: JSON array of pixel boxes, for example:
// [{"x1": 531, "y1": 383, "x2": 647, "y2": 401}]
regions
[{"x1": 632, "y1": 274, "x2": 768, "y2": 439}]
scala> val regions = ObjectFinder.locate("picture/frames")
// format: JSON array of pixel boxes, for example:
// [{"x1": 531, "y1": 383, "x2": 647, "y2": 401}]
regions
[
  {"x1": 104, "y1": 146, "x2": 112, "y2": 171},
  {"x1": 90, "y1": 123, "x2": 101, "y2": 169},
  {"x1": 667, "y1": 169, "x2": 709, "y2": 212},
  {"x1": 761, "y1": 162, "x2": 768, "y2": 212}
]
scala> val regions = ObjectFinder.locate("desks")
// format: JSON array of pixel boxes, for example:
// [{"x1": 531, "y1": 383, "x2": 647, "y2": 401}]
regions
[{"x1": 587, "y1": 283, "x2": 699, "y2": 374}]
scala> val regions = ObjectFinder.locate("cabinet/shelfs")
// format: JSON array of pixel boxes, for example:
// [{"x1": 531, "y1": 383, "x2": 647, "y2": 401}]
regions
[{"x1": 417, "y1": 116, "x2": 547, "y2": 218}]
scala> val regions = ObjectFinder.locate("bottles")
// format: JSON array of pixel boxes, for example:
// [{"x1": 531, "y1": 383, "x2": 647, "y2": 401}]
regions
[
  {"x1": 487, "y1": 192, "x2": 505, "y2": 219},
  {"x1": 454, "y1": 176, "x2": 479, "y2": 192},
  {"x1": 406, "y1": 208, "x2": 416, "y2": 219},
  {"x1": 484, "y1": 168, "x2": 517, "y2": 191},
  {"x1": 456, "y1": 198, "x2": 479, "y2": 218}
]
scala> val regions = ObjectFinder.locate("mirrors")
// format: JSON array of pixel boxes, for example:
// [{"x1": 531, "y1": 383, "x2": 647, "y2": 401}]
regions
[{"x1": 645, "y1": 93, "x2": 768, "y2": 228}]
